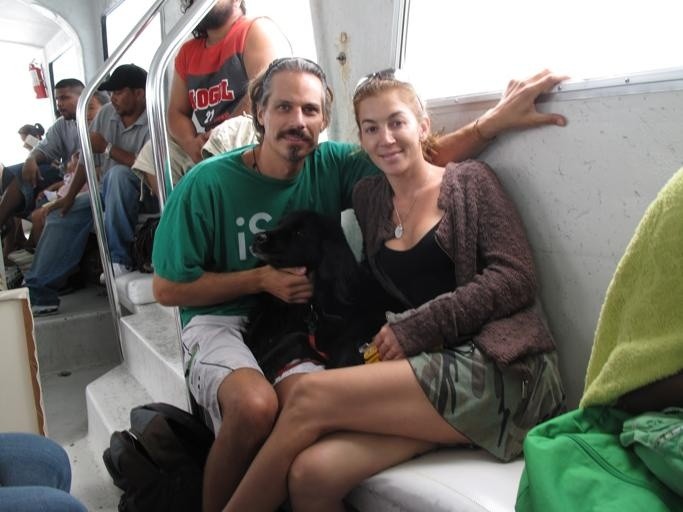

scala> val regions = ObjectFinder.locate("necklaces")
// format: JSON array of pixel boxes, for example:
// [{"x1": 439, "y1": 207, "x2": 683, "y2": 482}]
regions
[{"x1": 388, "y1": 179, "x2": 427, "y2": 244}]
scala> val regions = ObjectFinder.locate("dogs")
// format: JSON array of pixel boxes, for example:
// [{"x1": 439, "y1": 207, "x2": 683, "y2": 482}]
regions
[{"x1": 242, "y1": 206, "x2": 406, "y2": 383}]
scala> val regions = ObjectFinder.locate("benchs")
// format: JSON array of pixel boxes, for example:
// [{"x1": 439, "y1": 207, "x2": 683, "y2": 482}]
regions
[
  {"x1": 191, "y1": 348, "x2": 683, "y2": 512},
  {"x1": 81, "y1": 209, "x2": 161, "y2": 273}
]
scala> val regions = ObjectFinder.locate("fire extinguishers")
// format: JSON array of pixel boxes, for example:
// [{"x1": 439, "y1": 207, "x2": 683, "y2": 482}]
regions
[{"x1": 29, "y1": 58, "x2": 47, "y2": 98}]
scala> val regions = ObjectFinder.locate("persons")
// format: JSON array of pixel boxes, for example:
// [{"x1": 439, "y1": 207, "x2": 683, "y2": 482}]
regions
[
  {"x1": 0, "y1": 430, "x2": 88, "y2": 511},
  {"x1": 151, "y1": 56, "x2": 573, "y2": 511},
  {"x1": 1, "y1": 64, "x2": 164, "y2": 315},
  {"x1": 215, "y1": 65, "x2": 567, "y2": 511},
  {"x1": 155, "y1": 0, "x2": 291, "y2": 199}
]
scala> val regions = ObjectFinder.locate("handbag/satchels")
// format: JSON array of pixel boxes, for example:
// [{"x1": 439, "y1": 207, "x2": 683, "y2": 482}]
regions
[{"x1": 515, "y1": 406, "x2": 682, "y2": 512}]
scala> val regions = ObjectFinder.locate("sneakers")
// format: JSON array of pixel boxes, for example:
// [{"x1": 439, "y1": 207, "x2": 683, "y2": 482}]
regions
[
  {"x1": 99, "y1": 262, "x2": 134, "y2": 285},
  {"x1": 30, "y1": 303, "x2": 60, "y2": 317},
  {"x1": 5, "y1": 264, "x2": 23, "y2": 289}
]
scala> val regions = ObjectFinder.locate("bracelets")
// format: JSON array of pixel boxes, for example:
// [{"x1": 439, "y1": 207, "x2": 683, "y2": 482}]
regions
[{"x1": 471, "y1": 115, "x2": 498, "y2": 145}]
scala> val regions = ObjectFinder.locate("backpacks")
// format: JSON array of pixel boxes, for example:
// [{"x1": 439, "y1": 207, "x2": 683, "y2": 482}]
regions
[
  {"x1": 102, "y1": 403, "x2": 216, "y2": 512},
  {"x1": 130, "y1": 218, "x2": 161, "y2": 273}
]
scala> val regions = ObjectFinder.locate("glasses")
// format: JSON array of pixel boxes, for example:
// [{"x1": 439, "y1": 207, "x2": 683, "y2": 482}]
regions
[{"x1": 353, "y1": 68, "x2": 424, "y2": 111}]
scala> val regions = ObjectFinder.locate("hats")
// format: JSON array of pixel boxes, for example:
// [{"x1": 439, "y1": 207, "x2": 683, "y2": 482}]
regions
[{"x1": 97, "y1": 63, "x2": 147, "y2": 91}]
